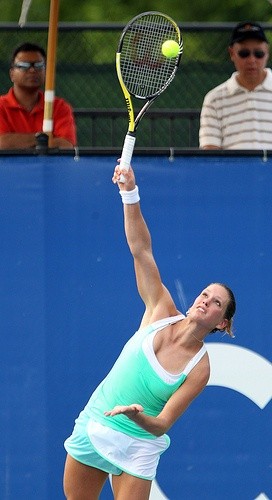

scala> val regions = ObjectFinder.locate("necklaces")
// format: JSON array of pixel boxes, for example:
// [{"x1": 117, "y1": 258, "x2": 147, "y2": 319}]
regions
[{"x1": 180, "y1": 318, "x2": 204, "y2": 343}]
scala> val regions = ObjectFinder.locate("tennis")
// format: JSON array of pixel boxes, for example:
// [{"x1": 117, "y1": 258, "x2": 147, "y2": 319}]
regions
[{"x1": 162, "y1": 40, "x2": 180, "y2": 57}]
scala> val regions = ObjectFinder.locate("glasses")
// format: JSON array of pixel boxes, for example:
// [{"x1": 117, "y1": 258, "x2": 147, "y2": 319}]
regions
[
  {"x1": 238, "y1": 48, "x2": 266, "y2": 59},
  {"x1": 12, "y1": 61, "x2": 46, "y2": 72}
]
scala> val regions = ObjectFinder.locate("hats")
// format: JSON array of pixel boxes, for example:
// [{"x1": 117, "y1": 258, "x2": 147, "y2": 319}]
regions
[{"x1": 231, "y1": 21, "x2": 267, "y2": 43}]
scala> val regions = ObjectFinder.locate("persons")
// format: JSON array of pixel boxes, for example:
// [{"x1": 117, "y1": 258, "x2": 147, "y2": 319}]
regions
[
  {"x1": 62, "y1": 158, "x2": 237, "y2": 500},
  {"x1": 0, "y1": 42, "x2": 78, "y2": 150},
  {"x1": 198, "y1": 21, "x2": 272, "y2": 150}
]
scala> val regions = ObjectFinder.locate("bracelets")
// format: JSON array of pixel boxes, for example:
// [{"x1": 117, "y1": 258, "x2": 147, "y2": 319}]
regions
[{"x1": 117, "y1": 186, "x2": 142, "y2": 205}]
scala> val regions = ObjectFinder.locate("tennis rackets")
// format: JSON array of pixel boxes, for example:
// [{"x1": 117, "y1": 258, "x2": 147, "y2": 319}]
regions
[{"x1": 115, "y1": 11, "x2": 183, "y2": 184}]
